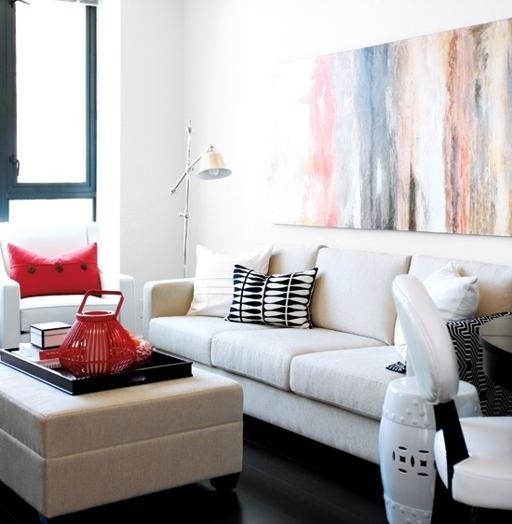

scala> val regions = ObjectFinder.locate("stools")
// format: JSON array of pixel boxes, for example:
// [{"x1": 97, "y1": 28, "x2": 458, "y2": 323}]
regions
[{"x1": 378, "y1": 375, "x2": 481, "y2": 523}]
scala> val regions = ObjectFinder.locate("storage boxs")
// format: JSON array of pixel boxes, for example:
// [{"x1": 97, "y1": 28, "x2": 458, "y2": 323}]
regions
[{"x1": 28, "y1": 320, "x2": 73, "y2": 349}]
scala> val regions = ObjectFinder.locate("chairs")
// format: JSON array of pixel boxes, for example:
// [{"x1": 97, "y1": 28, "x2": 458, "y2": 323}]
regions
[{"x1": 391, "y1": 272, "x2": 511, "y2": 523}]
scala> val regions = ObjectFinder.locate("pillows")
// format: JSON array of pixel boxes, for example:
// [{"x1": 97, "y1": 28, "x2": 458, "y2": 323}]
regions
[
  {"x1": 5, "y1": 242, "x2": 103, "y2": 298},
  {"x1": 186, "y1": 242, "x2": 277, "y2": 318},
  {"x1": 386, "y1": 311, "x2": 511, "y2": 415},
  {"x1": 225, "y1": 263, "x2": 318, "y2": 330},
  {"x1": 394, "y1": 262, "x2": 480, "y2": 363}
]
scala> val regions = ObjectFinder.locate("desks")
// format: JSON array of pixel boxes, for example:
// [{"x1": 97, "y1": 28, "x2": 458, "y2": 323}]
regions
[{"x1": 478, "y1": 313, "x2": 512, "y2": 391}]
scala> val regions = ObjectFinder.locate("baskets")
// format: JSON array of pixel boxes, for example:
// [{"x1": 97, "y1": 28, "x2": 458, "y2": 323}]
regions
[{"x1": 59, "y1": 290, "x2": 136, "y2": 377}]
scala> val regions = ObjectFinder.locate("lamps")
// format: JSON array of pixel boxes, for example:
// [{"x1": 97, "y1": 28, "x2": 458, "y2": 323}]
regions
[{"x1": 168, "y1": 117, "x2": 233, "y2": 277}]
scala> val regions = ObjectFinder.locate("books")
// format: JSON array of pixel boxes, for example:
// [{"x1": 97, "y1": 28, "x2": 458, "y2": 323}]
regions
[{"x1": 12, "y1": 340, "x2": 86, "y2": 368}]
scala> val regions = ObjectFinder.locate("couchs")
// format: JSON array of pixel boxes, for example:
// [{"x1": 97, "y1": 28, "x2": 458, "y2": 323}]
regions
[
  {"x1": 142, "y1": 224, "x2": 512, "y2": 464},
  {"x1": 0, "y1": 223, "x2": 136, "y2": 349}
]
